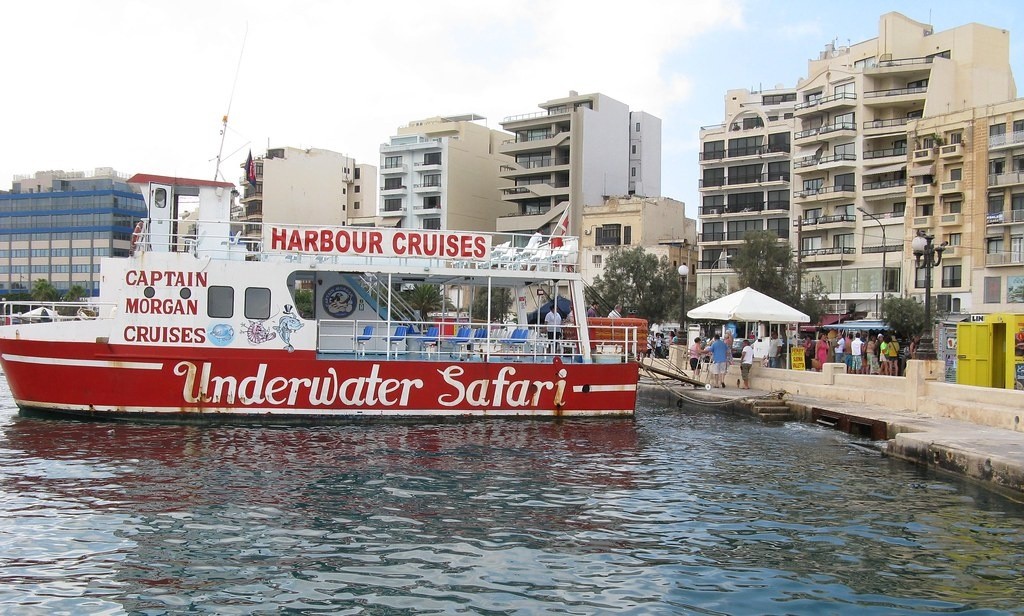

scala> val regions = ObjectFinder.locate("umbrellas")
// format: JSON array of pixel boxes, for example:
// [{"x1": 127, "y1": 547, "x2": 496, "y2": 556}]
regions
[{"x1": 14, "y1": 306, "x2": 64, "y2": 322}]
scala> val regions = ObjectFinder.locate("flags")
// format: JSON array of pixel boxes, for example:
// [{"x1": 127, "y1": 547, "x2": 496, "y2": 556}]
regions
[
  {"x1": 558, "y1": 205, "x2": 569, "y2": 236},
  {"x1": 245, "y1": 150, "x2": 256, "y2": 188}
]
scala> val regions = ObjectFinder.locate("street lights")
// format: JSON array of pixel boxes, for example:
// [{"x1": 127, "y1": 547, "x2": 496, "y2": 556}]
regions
[
  {"x1": 913, "y1": 232, "x2": 950, "y2": 358},
  {"x1": 710, "y1": 254, "x2": 732, "y2": 301},
  {"x1": 857, "y1": 207, "x2": 886, "y2": 318},
  {"x1": 676, "y1": 265, "x2": 689, "y2": 345}
]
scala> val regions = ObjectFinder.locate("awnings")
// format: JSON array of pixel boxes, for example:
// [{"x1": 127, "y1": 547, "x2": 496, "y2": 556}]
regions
[
  {"x1": 378, "y1": 217, "x2": 403, "y2": 227},
  {"x1": 909, "y1": 163, "x2": 936, "y2": 177},
  {"x1": 860, "y1": 163, "x2": 908, "y2": 176},
  {"x1": 785, "y1": 314, "x2": 848, "y2": 332},
  {"x1": 792, "y1": 143, "x2": 825, "y2": 160},
  {"x1": 823, "y1": 319, "x2": 894, "y2": 331}
]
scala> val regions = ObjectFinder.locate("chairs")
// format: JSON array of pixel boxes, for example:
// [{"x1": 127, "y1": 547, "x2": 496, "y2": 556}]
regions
[
  {"x1": 351, "y1": 326, "x2": 537, "y2": 362},
  {"x1": 490, "y1": 240, "x2": 571, "y2": 272}
]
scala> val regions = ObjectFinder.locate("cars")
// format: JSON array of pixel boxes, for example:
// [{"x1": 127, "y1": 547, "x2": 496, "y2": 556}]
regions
[{"x1": 662, "y1": 329, "x2": 687, "y2": 352}]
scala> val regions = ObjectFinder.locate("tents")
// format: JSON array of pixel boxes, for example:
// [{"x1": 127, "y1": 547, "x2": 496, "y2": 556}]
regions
[
  {"x1": 520, "y1": 295, "x2": 576, "y2": 325},
  {"x1": 686, "y1": 286, "x2": 811, "y2": 369}
]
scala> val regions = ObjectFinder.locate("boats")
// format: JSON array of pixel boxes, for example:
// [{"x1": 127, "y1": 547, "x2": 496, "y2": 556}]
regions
[{"x1": 0, "y1": 171, "x2": 649, "y2": 418}]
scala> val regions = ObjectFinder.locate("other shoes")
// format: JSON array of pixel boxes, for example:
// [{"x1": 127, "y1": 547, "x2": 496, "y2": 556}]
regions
[
  {"x1": 713, "y1": 386, "x2": 720, "y2": 388},
  {"x1": 721, "y1": 382, "x2": 725, "y2": 388}
]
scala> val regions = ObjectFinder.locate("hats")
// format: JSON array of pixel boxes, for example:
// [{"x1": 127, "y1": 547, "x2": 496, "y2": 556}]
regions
[
  {"x1": 891, "y1": 335, "x2": 897, "y2": 342},
  {"x1": 877, "y1": 334, "x2": 884, "y2": 338}
]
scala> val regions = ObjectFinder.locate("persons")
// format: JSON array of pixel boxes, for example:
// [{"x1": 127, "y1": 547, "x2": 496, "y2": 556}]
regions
[
  {"x1": 814, "y1": 333, "x2": 829, "y2": 372},
  {"x1": 647, "y1": 330, "x2": 677, "y2": 358},
  {"x1": 543, "y1": 305, "x2": 562, "y2": 353},
  {"x1": 578, "y1": 301, "x2": 599, "y2": 363},
  {"x1": 608, "y1": 303, "x2": 623, "y2": 318},
  {"x1": 767, "y1": 331, "x2": 782, "y2": 368},
  {"x1": 723, "y1": 330, "x2": 735, "y2": 366},
  {"x1": 750, "y1": 331, "x2": 755, "y2": 339},
  {"x1": 834, "y1": 330, "x2": 919, "y2": 376},
  {"x1": 690, "y1": 337, "x2": 702, "y2": 381},
  {"x1": 802, "y1": 336, "x2": 813, "y2": 370},
  {"x1": 702, "y1": 334, "x2": 728, "y2": 388},
  {"x1": 567, "y1": 302, "x2": 574, "y2": 321},
  {"x1": 740, "y1": 340, "x2": 754, "y2": 389},
  {"x1": 522, "y1": 228, "x2": 544, "y2": 259}
]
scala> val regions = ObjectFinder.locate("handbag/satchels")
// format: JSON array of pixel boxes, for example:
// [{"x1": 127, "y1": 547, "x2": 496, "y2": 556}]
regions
[{"x1": 811, "y1": 359, "x2": 820, "y2": 368}]
